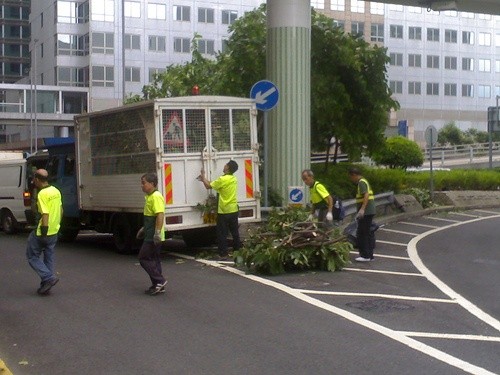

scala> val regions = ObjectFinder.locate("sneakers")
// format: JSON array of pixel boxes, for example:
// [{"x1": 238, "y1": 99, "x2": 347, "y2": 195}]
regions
[
  {"x1": 145, "y1": 285, "x2": 165, "y2": 293},
  {"x1": 150, "y1": 280, "x2": 167, "y2": 295},
  {"x1": 38, "y1": 276, "x2": 58, "y2": 294},
  {"x1": 355, "y1": 256, "x2": 371, "y2": 262}
]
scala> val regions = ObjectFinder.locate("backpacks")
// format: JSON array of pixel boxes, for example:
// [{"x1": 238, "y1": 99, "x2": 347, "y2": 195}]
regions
[{"x1": 331, "y1": 194, "x2": 345, "y2": 219}]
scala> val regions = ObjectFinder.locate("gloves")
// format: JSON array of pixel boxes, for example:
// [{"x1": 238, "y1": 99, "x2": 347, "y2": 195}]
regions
[{"x1": 356, "y1": 209, "x2": 365, "y2": 218}]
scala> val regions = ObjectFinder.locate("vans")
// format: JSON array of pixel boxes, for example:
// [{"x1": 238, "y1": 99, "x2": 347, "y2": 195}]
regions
[{"x1": 0, "y1": 150, "x2": 29, "y2": 236}]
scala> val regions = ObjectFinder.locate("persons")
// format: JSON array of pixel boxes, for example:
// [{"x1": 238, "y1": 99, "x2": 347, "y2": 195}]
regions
[
  {"x1": 350, "y1": 167, "x2": 377, "y2": 261},
  {"x1": 302, "y1": 169, "x2": 334, "y2": 228},
  {"x1": 26, "y1": 169, "x2": 63, "y2": 295},
  {"x1": 136, "y1": 173, "x2": 167, "y2": 295},
  {"x1": 201, "y1": 160, "x2": 242, "y2": 260}
]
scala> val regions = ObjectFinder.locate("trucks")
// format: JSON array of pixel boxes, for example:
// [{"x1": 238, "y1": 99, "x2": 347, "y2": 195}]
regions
[{"x1": 23, "y1": 96, "x2": 262, "y2": 256}]
